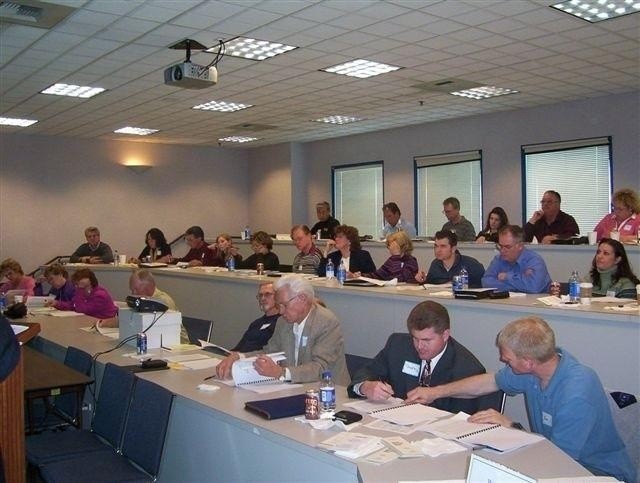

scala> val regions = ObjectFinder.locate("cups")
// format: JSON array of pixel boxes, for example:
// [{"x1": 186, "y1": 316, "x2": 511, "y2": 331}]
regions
[
  {"x1": 610, "y1": 230, "x2": 619, "y2": 241},
  {"x1": 120, "y1": 254, "x2": 126, "y2": 263},
  {"x1": 588, "y1": 232, "x2": 597, "y2": 246},
  {"x1": 15, "y1": 295, "x2": 23, "y2": 303},
  {"x1": 579, "y1": 282, "x2": 593, "y2": 308},
  {"x1": 636, "y1": 285, "x2": 640, "y2": 305},
  {"x1": 316, "y1": 232, "x2": 321, "y2": 240},
  {"x1": 240, "y1": 231, "x2": 245, "y2": 241}
]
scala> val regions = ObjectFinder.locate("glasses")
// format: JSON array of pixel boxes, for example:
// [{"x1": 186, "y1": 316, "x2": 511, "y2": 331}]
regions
[
  {"x1": 384, "y1": 215, "x2": 395, "y2": 219},
  {"x1": 275, "y1": 295, "x2": 300, "y2": 309},
  {"x1": 334, "y1": 235, "x2": 348, "y2": 239},
  {"x1": 443, "y1": 210, "x2": 457, "y2": 213},
  {"x1": 612, "y1": 206, "x2": 630, "y2": 211},
  {"x1": 496, "y1": 243, "x2": 519, "y2": 249},
  {"x1": 540, "y1": 201, "x2": 560, "y2": 205},
  {"x1": 256, "y1": 293, "x2": 276, "y2": 300}
]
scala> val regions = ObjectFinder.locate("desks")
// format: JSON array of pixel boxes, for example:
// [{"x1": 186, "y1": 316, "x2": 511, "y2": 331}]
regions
[
  {"x1": 1, "y1": 303, "x2": 595, "y2": 483},
  {"x1": 201, "y1": 236, "x2": 640, "y2": 291},
  {"x1": 40, "y1": 263, "x2": 640, "y2": 456}
]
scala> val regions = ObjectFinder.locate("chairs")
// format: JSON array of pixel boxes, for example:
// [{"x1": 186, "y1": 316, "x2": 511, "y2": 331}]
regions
[
  {"x1": 38, "y1": 376, "x2": 176, "y2": 483},
  {"x1": 182, "y1": 316, "x2": 213, "y2": 346},
  {"x1": 478, "y1": 389, "x2": 507, "y2": 416},
  {"x1": 24, "y1": 362, "x2": 136, "y2": 466}
]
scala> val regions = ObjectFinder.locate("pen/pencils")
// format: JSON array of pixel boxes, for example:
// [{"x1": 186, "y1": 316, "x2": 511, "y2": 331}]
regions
[
  {"x1": 560, "y1": 301, "x2": 578, "y2": 304},
  {"x1": 45, "y1": 299, "x2": 52, "y2": 309},
  {"x1": 89, "y1": 318, "x2": 102, "y2": 330},
  {"x1": 204, "y1": 372, "x2": 217, "y2": 381},
  {"x1": 421, "y1": 266, "x2": 424, "y2": 283},
  {"x1": 380, "y1": 376, "x2": 389, "y2": 388},
  {"x1": 472, "y1": 444, "x2": 489, "y2": 451}
]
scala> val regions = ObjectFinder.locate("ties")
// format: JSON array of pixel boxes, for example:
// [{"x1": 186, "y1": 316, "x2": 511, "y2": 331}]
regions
[{"x1": 419, "y1": 360, "x2": 431, "y2": 387}]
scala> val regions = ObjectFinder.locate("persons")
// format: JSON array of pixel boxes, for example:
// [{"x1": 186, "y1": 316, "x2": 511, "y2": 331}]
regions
[
  {"x1": 32, "y1": 263, "x2": 75, "y2": 301},
  {"x1": 311, "y1": 202, "x2": 341, "y2": 239},
  {"x1": 481, "y1": 226, "x2": 553, "y2": 295},
  {"x1": 49, "y1": 270, "x2": 118, "y2": 318},
  {"x1": 71, "y1": 226, "x2": 112, "y2": 265},
  {"x1": 347, "y1": 300, "x2": 495, "y2": 417},
  {"x1": 475, "y1": 207, "x2": 509, "y2": 244},
  {"x1": 584, "y1": 238, "x2": 637, "y2": 299},
  {"x1": 402, "y1": 319, "x2": 640, "y2": 483},
  {"x1": 379, "y1": 201, "x2": 416, "y2": 239},
  {"x1": 522, "y1": 190, "x2": 580, "y2": 244},
  {"x1": 594, "y1": 188, "x2": 640, "y2": 242},
  {"x1": 94, "y1": 270, "x2": 192, "y2": 344},
  {"x1": 168, "y1": 225, "x2": 217, "y2": 267},
  {"x1": 215, "y1": 273, "x2": 352, "y2": 386},
  {"x1": 128, "y1": 228, "x2": 172, "y2": 265},
  {"x1": 441, "y1": 197, "x2": 475, "y2": 241},
  {"x1": 227, "y1": 232, "x2": 280, "y2": 272},
  {"x1": 0, "y1": 257, "x2": 35, "y2": 296},
  {"x1": 319, "y1": 225, "x2": 376, "y2": 282},
  {"x1": 415, "y1": 230, "x2": 484, "y2": 290},
  {"x1": 292, "y1": 224, "x2": 323, "y2": 275},
  {"x1": 353, "y1": 230, "x2": 418, "y2": 286},
  {"x1": 203, "y1": 234, "x2": 242, "y2": 269},
  {"x1": 229, "y1": 280, "x2": 325, "y2": 355}
]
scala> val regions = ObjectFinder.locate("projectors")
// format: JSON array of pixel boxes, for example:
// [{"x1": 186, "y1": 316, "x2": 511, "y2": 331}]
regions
[
  {"x1": 164, "y1": 64, "x2": 216, "y2": 89},
  {"x1": 126, "y1": 295, "x2": 168, "y2": 313}
]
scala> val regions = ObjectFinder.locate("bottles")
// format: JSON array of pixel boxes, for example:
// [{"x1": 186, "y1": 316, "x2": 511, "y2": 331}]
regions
[
  {"x1": 452, "y1": 274, "x2": 462, "y2": 291},
  {"x1": 460, "y1": 265, "x2": 469, "y2": 288},
  {"x1": 228, "y1": 256, "x2": 234, "y2": 270},
  {"x1": 114, "y1": 249, "x2": 120, "y2": 267},
  {"x1": 549, "y1": 280, "x2": 561, "y2": 297},
  {"x1": 337, "y1": 259, "x2": 347, "y2": 286},
  {"x1": 326, "y1": 258, "x2": 334, "y2": 277},
  {"x1": 256, "y1": 263, "x2": 264, "y2": 275},
  {"x1": 0, "y1": 292, "x2": 7, "y2": 312},
  {"x1": 303, "y1": 387, "x2": 321, "y2": 421},
  {"x1": 319, "y1": 370, "x2": 337, "y2": 410},
  {"x1": 569, "y1": 269, "x2": 582, "y2": 302},
  {"x1": 136, "y1": 331, "x2": 148, "y2": 355}
]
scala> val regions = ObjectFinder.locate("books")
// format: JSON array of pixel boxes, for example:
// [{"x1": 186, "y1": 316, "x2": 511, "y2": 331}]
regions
[
  {"x1": 344, "y1": 395, "x2": 452, "y2": 426},
  {"x1": 216, "y1": 351, "x2": 286, "y2": 385},
  {"x1": 112, "y1": 304, "x2": 187, "y2": 351},
  {"x1": 46, "y1": 309, "x2": 83, "y2": 318},
  {"x1": 423, "y1": 412, "x2": 545, "y2": 455},
  {"x1": 345, "y1": 279, "x2": 377, "y2": 287},
  {"x1": 97, "y1": 320, "x2": 119, "y2": 342},
  {"x1": 246, "y1": 392, "x2": 309, "y2": 420},
  {"x1": 455, "y1": 288, "x2": 493, "y2": 299}
]
